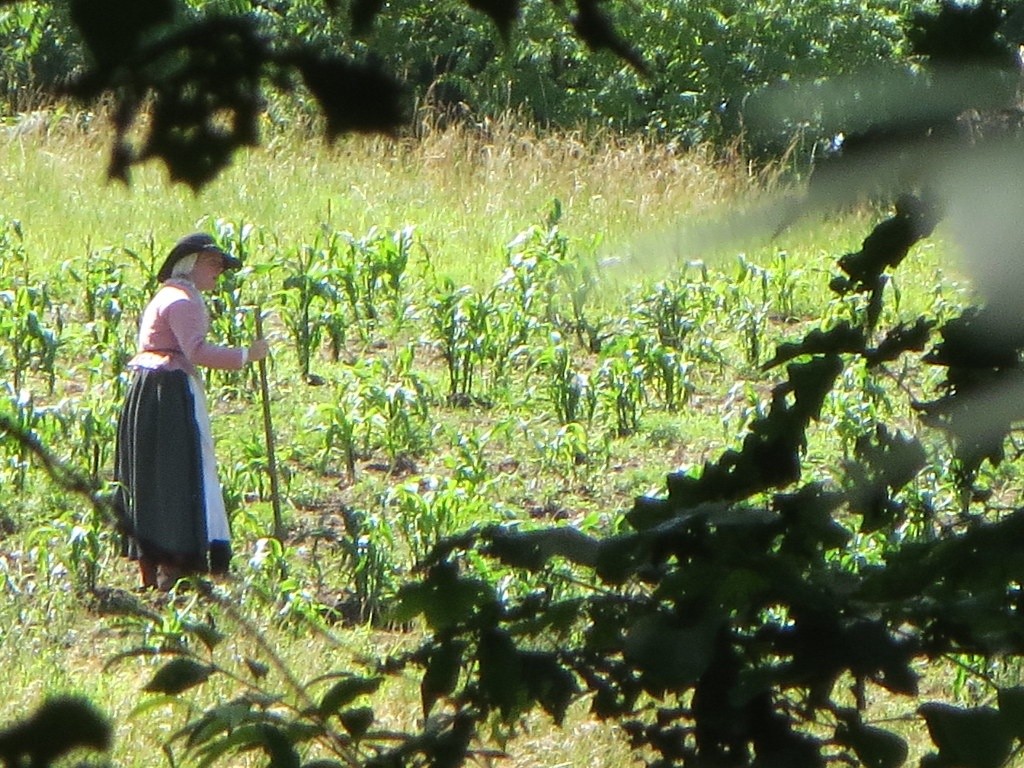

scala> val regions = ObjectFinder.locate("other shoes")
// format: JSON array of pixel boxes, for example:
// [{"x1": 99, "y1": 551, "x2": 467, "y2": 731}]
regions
[
  {"x1": 159, "y1": 575, "x2": 210, "y2": 592},
  {"x1": 138, "y1": 580, "x2": 157, "y2": 590}
]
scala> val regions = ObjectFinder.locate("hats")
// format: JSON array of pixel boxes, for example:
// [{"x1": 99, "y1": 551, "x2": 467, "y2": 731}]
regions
[{"x1": 158, "y1": 234, "x2": 242, "y2": 282}]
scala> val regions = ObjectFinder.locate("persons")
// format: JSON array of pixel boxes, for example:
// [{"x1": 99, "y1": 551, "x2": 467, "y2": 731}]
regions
[{"x1": 115, "y1": 232, "x2": 271, "y2": 592}]
